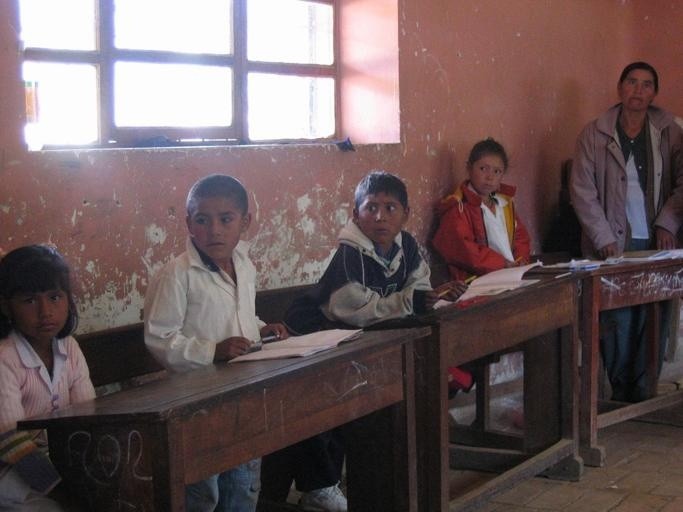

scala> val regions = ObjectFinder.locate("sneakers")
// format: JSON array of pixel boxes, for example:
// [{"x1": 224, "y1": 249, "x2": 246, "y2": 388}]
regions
[{"x1": 296, "y1": 486, "x2": 347, "y2": 512}]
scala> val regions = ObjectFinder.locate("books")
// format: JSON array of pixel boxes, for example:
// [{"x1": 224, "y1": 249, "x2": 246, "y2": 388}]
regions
[
  {"x1": 620, "y1": 249, "x2": 663, "y2": 261},
  {"x1": 225, "y1": 329, "x2": 364, "y2": 365},
  {"x1": 432, "y1": 259, "x2": 544, "y2": 309},
  {"x1": 548, "y1": 259, "x2": 604, "y2": 270},
  {"x1": 654, "y1": 250, "x2": 670, "y2": 259},
  {"x1": 662, "y1": 249, "x2": 682, "y2": 259}
]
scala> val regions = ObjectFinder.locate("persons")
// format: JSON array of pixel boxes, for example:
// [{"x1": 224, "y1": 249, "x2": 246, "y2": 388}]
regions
[
  {"x1": 0, "y1": 244, "x2": 100, "y2": 511},
  {"x1": 568, "y1": 60, "x2": 683, "y2": 404},
  {"x1": 427, "y1": 135, "x2": 531, "y2": 400},
  {"x1": 142, "y1": 171, "x2": 291, "y2": 512},
  {"x1": 256, "y1": 169, "x2": 469, "y2": 512}
]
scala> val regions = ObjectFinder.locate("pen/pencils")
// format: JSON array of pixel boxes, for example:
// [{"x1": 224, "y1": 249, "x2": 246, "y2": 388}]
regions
[
  {"x1": 261, "y1": 335, "x2": 281, "y2": 342},
  {"x1": 515, "y1": 256, "x2": 523, "y2": 262},
  {"x1": 554, "y1": 271, "x2": 572, "y2": 279},
  {"x1": 437, "y1": 275, "x2": 477, "y2": 298}
]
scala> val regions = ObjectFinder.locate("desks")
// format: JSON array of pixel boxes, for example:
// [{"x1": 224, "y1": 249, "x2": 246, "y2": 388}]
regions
[
  {"x1": 21, "y1": 322, "x2": 435, "y2": 511},
  {"x1": 520, "y1": 252, "x2": 682, "y2": 466},
  {"x1": 362, "y1": 264, "x2": 591, "y2": 510}
]
